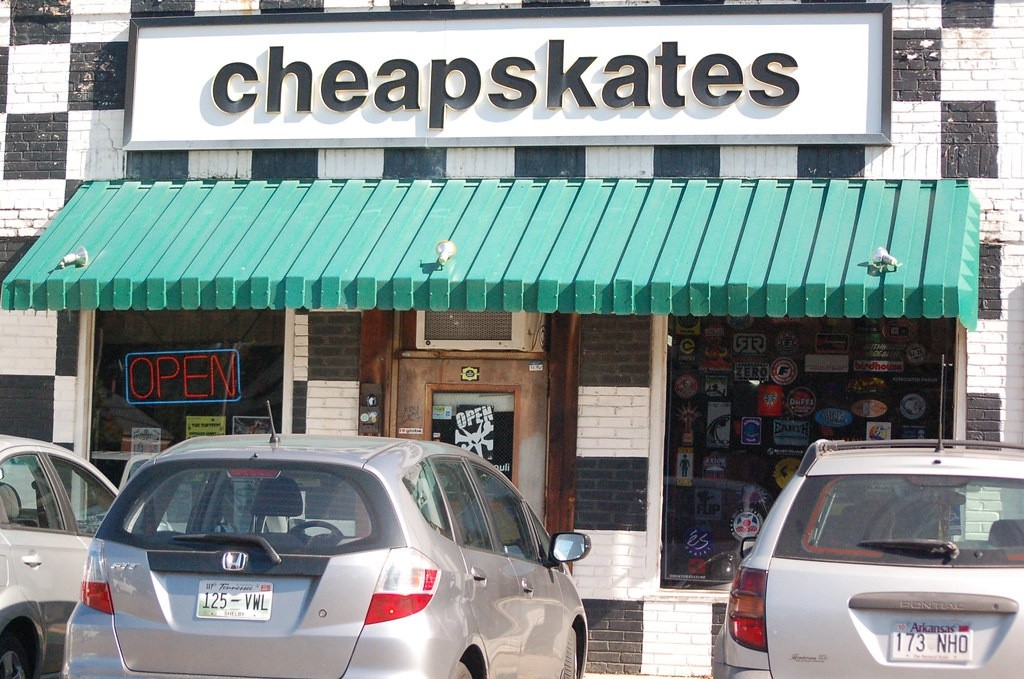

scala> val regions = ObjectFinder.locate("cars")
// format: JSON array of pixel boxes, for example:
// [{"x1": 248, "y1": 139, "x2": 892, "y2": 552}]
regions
[
  {"x1": 0, "y1": 436, "x2": 173, "y2": 678},
  {"x1": 62, "y1": 431, "x2": 592, "y2": 679},
  {"x1": 711, "y1": 438, "x2": 1023, "y2": 679}
]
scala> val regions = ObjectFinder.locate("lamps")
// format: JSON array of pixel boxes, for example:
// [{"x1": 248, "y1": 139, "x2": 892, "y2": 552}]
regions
[
  {"x1": 436, "y1": 241, "x2": 457, "y2": 264},
  {"x1": 60, "y1": 246, "x2": 88, "y2": 268},
  {"x1": 871, "y1": 247, "x2": 899, "y2": 270}
]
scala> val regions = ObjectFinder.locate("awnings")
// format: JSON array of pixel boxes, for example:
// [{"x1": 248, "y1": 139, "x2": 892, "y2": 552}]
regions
[{"x1": 2, "y1": 175, "x2": 980, "y2": 332}]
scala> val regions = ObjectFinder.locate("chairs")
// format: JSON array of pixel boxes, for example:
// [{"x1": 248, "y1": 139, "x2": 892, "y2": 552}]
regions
[{"x1": 0, "y1": 483, "x2": 40, "y2": 527}]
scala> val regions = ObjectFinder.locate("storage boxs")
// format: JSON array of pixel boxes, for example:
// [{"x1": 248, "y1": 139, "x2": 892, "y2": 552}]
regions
[{"x1": 119, "y1": 436, "x2": 170, "y2": 453}]
